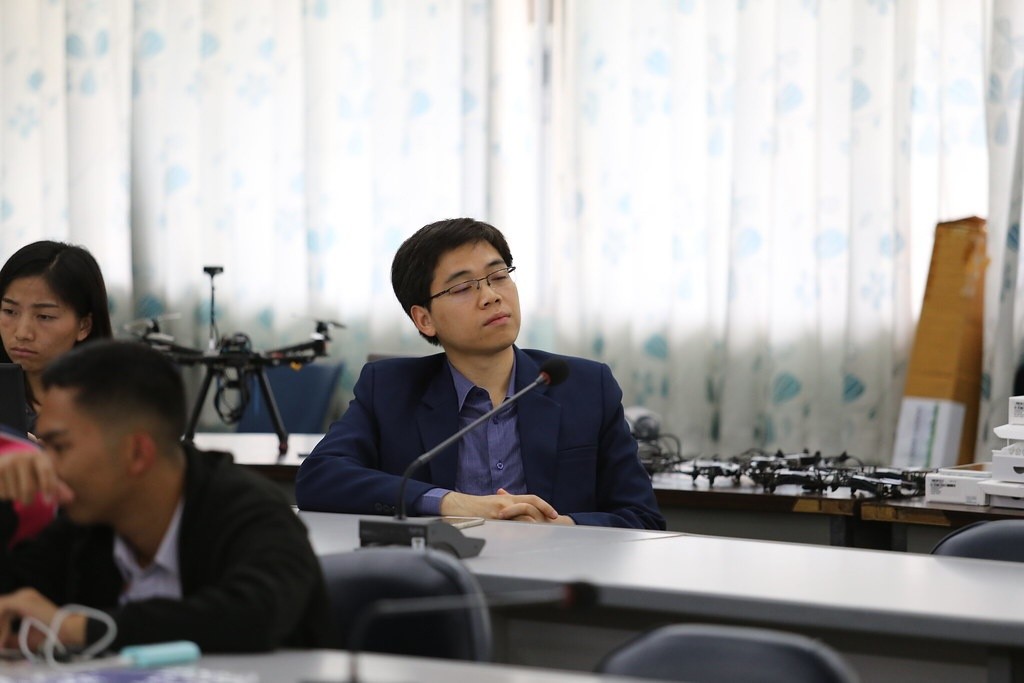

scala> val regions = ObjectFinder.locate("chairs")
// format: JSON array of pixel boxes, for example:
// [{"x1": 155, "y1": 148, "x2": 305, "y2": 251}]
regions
[
  {"x1": 239, "y1": 360, "x2": 343, "y2": 434},
  {"x1": 314, "y1": 517, "x2": 1024, "y2": 683}
]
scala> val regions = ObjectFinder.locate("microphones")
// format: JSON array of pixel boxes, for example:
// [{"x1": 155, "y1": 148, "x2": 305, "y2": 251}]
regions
[{"x1": 358, "y1": 357, "x2": 569, "y2": 560}]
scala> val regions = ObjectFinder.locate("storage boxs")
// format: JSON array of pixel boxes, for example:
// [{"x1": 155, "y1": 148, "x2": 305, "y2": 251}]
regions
[
  {"x1": 926, "y1": 395, "x2": 1024, "y2": 510},
  {"x1": 895, "y1": 217, "x2": 989, "y2": 472}
]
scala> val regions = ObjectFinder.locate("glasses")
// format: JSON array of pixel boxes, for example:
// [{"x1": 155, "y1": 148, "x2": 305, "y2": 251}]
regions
[{"x1": 428, "y1": 266, "x2": 518, "y2": 300}]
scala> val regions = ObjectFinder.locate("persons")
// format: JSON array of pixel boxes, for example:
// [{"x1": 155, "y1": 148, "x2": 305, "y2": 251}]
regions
[
  {"x1": 0, "y1": 241, "x2": 319, "y2": 658},
  {"x1": 294, "y1": 218, "x2": 667, "y2": 532}
]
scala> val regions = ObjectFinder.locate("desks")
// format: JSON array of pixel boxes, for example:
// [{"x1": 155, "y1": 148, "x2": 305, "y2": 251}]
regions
[
  {"x1": 178, "y1": 431, "x2": 1024, "y2": 555},
  {"x1": 290, "y1": 503, "x2": 1024, "y2": 683}
]
